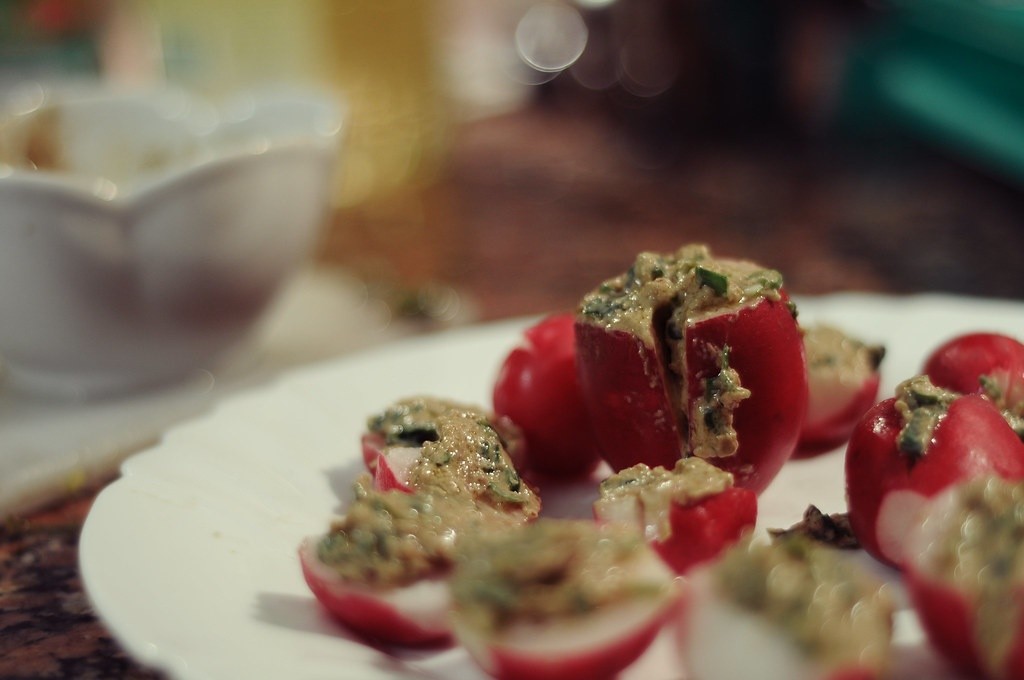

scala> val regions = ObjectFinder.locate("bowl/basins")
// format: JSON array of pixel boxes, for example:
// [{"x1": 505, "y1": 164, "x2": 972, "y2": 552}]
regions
[{"x1": 0, "y1": 76, "x2": 349, "y2": 405}]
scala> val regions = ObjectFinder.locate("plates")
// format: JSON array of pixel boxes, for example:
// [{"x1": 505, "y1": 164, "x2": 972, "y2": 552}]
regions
[{"x1": 77, "y1": 291, "x2": 1024, "y2": 680}]
